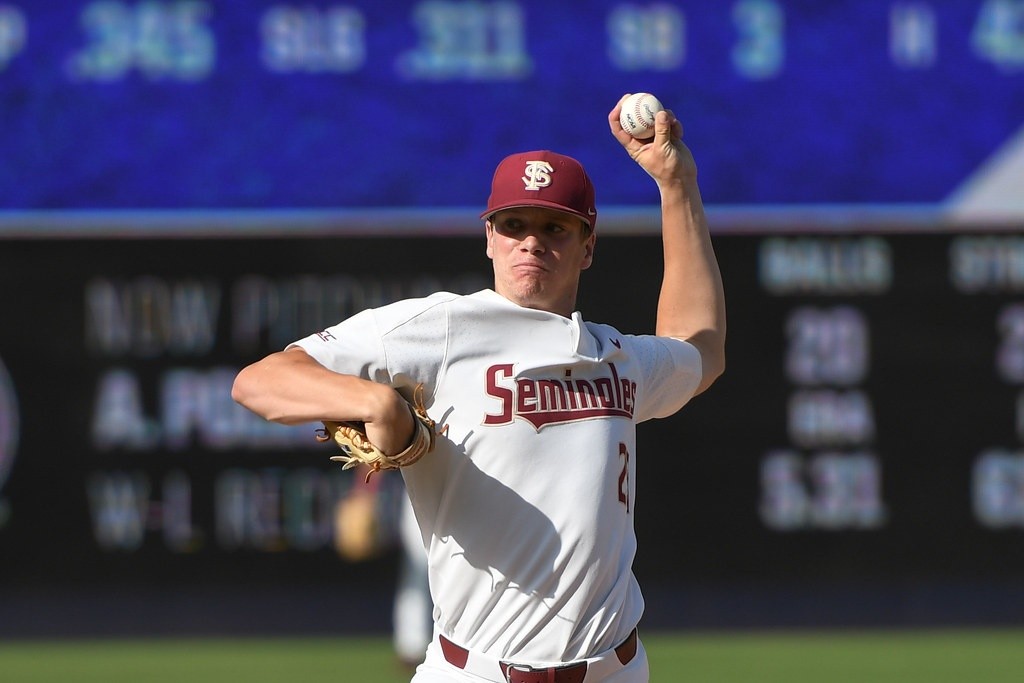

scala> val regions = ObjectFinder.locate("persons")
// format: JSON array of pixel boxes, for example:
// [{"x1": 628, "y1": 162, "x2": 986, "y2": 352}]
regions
[{"x1": 231, "y1": 93, "x2": 728, "y2": 683}]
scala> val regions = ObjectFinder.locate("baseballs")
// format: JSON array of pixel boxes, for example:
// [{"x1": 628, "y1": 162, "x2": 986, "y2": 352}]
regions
[{"x1": 619, "y1": 90, "x2": 666, "y2": 140}]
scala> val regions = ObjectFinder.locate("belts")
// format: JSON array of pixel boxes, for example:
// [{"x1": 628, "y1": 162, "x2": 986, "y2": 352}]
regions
[{"x1": 439, "y1": 626, "x2": 638, "y2": 683}]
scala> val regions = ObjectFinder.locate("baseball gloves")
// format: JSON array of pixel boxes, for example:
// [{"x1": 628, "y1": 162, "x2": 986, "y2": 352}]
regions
[{"x1": 314, "y1": 381, "x2": 449, "y2": 486}]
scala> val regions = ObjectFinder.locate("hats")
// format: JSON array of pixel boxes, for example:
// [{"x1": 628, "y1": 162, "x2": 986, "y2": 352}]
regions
[{"x1": 479, "y1": 150, "x2": 597, "y2": 231}]
